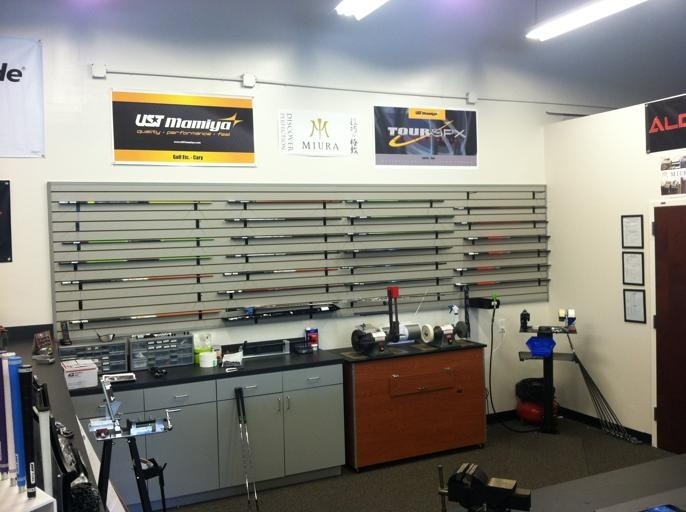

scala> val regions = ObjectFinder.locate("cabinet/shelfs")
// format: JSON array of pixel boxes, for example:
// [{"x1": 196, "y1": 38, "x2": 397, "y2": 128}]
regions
[
  {"x1": 327, "y1": 341, "x2": 491, "y2": 475},
  {"x1": 218, "y1": 364, "x2": 343, "y2": 501},
  {"x1": 72, "y1": 381, "x2": 222, "y2": 512}
]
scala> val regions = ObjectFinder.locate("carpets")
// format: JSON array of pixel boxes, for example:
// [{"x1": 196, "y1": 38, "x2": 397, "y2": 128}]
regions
[{"x1": 157, "y1": 411, "x2": 682, "y2": 510}]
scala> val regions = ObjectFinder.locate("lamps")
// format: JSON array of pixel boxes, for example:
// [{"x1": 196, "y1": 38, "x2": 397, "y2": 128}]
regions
[
  {"x1": 524, "y1": 0, "x2": 650, "y2": 44},
  {"x1": 334, "y1": 0, "x2": 389, "y2": 24}
]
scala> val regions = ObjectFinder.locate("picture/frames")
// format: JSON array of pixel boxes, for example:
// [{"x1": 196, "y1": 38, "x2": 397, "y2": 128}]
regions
[{"x1": 619, "y1": 215, "x2": 647, "y2": 325}]
